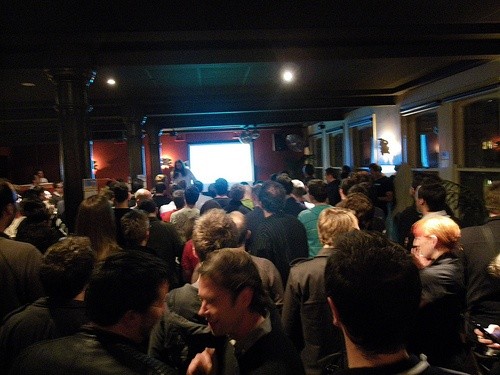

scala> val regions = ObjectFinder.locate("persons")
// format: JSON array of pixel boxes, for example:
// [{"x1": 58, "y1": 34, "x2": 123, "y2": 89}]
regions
[
  {"x1": 0, "y1": 180, "x2": 45, "y2": 320},
  {"x1": 186, "y1": 247, "x2": 307, "y2": 375},
  {"x1": 0, "y1": 235, "x2": 97, "y2": 375},
  {"x1": 318, "y1": 230, "x2": 473, "y2": 375},
  {"x1": 0, "y1": 160, "x2": 500, "y2": 290},
  {"x1": 147, "y1": 207, "x2": 240, "y2": 375},
  {"x1": 280, "y1": 207, "x2": 360, "y2": 375},
  {"x1": 8, "y1": 250, "x2": 179, "y2": 375},
  {"x1": 411, "y1": 214, "x2": 500, "y2": 367}
]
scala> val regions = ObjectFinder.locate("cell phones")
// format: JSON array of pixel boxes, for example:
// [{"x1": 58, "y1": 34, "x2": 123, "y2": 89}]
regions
[{"x1": 472, "y1": 321, "x2": 500, "y2": 344}]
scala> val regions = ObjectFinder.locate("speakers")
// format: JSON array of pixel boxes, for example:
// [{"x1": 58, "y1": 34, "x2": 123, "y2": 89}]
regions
[{"x1": 272, "y1": 134, "x2": 287, "y2": 151}]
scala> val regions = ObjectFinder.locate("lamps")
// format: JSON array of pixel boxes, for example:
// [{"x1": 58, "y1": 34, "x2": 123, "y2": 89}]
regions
[
  {"x1": 62, "y1": 64, "x2": 96, "y2": 89},
  {"x1": 239, "y1": 124, "x2": 261, "y2": 145}
]
